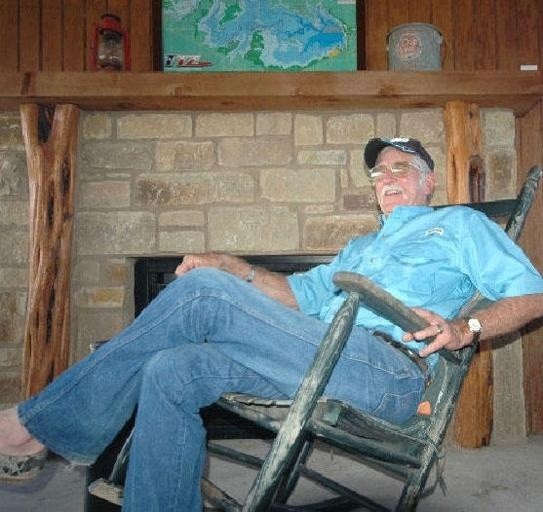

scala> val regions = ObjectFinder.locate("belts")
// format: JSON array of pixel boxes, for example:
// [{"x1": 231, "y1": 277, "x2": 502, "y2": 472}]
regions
[{"x1": 359, "y1": 325, "x2": 431, "y2": 389}]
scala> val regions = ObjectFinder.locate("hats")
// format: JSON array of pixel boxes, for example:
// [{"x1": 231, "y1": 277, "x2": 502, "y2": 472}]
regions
[{"x1": 364, "y1": 137, "x2": 435, "y2": 171}]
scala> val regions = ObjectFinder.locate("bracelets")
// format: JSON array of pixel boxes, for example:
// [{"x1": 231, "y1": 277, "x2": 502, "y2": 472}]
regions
[{"x1": 245, "y1": 264, "x2": 256, "y2": 282}]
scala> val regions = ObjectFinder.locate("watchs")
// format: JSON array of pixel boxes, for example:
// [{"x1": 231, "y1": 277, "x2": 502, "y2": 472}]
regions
[{"x1": 462, "y1": 315, "x2": 482, "y2": 346}]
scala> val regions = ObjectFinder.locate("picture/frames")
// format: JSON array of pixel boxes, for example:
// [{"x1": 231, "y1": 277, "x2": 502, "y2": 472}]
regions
[{"x1": 149, "y1": 1, "x2": 367, "y2": 73}]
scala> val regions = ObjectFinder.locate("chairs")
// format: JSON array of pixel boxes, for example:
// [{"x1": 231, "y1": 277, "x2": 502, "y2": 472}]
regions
[{"x1": 77, "y1": 157, "x2": 542, "y2": 511}]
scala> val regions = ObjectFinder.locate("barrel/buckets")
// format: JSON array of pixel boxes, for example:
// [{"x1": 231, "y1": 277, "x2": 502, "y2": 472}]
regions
[{"x1": 384, "y1": 23, "x2": 448, "y2": 71}]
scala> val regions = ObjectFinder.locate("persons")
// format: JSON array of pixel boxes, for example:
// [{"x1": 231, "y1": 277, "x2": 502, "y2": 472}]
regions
[{"x1": 1, "y1": 133, "x2": 541, "y2": 512}]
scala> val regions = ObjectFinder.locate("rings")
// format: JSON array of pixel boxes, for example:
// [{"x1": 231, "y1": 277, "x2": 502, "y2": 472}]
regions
[{"x1": 433, "y1": 322, "x2": 445, "y2": 336}]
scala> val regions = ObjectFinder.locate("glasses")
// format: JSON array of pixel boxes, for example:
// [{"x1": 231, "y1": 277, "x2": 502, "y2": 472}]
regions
[{"x1": 369, "y1": 161, "x2": 412, "y2": 180}]
